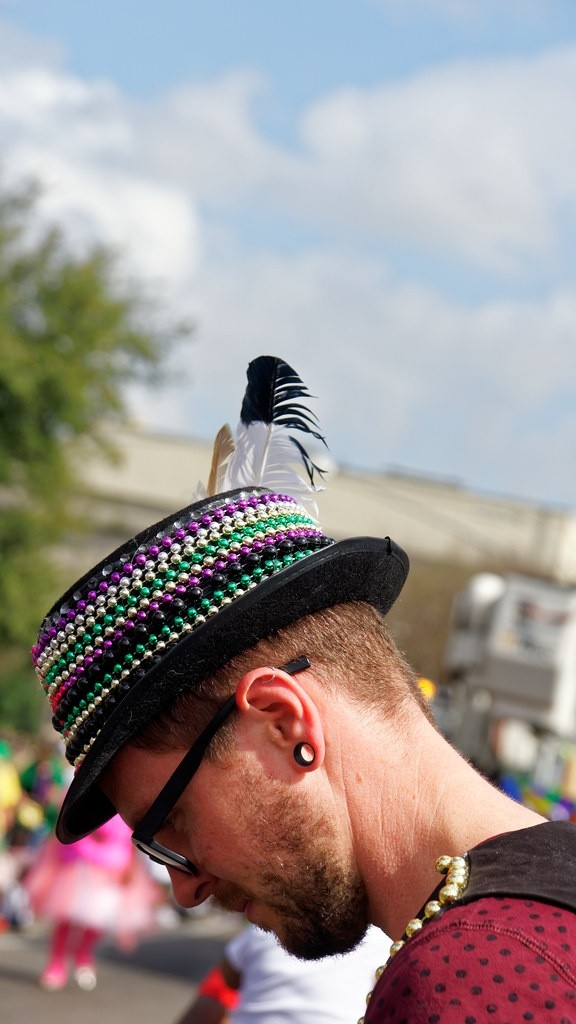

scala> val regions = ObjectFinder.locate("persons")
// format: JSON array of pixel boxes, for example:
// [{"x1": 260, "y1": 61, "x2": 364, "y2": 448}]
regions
[
  {"x1": 0, "y1": 719, "x2": 575, "y2": 1024},
  {"x1": 29, "y1": 355, "x2": 576, "y2": 1023}
]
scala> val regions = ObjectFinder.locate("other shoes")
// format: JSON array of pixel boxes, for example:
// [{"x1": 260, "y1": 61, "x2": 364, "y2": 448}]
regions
[
  {"x1": 74, "y1": 965, "x2": 96, "y2": 990},
  {"x1": 37, "y1": 964, "x2": 69, "y2": 992}
]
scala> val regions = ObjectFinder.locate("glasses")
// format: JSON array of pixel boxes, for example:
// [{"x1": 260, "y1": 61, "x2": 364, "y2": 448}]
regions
[{"x1": 129, "y1": 654, "x2": 312, "y2": 876}]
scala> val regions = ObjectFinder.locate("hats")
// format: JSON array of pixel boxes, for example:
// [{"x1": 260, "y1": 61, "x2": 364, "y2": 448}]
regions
[{"x1": 29, "y1": 485, "x2": 409, "y2": 843}]
